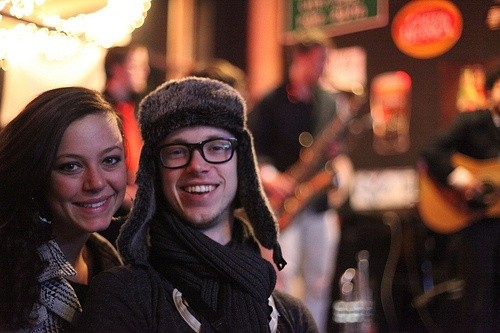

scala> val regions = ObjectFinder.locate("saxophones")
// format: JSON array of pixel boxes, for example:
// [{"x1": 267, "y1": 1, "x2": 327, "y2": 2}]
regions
[{"x1": 269, "y1": 81, "x2": 364, "y2": 230}]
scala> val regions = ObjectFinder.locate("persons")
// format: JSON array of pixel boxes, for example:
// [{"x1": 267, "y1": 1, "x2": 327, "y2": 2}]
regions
[
  {"x1": 98, "y1": 43, "x2": 249, "y2": 199},
  {"x1": 253, "y1": 30, "x2": 352, "y2": 333},
  {"x1": 81, "y1": 81, "x2": 318, "y2": 332},
  {"x1": 415, "y1": 69, "x2": 500, "y2": 332},
  {"x1": 1, "y1": 87, "x2": 128, "y2": 332}
]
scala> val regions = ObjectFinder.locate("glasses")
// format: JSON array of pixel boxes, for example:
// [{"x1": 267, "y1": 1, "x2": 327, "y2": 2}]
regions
[{"x1": 155, "y1": 137, "x2": 239, "y2": 169}]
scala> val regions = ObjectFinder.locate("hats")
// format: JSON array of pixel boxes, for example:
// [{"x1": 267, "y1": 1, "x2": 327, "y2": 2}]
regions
[{"x1": 116, "y1": 77, "x2": 287, "y2": 271}]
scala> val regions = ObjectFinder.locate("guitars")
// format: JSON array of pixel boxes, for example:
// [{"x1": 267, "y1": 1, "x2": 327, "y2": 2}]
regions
[{"x1": 417, "y1": 154, "x2": 500, "y2": 232}]
